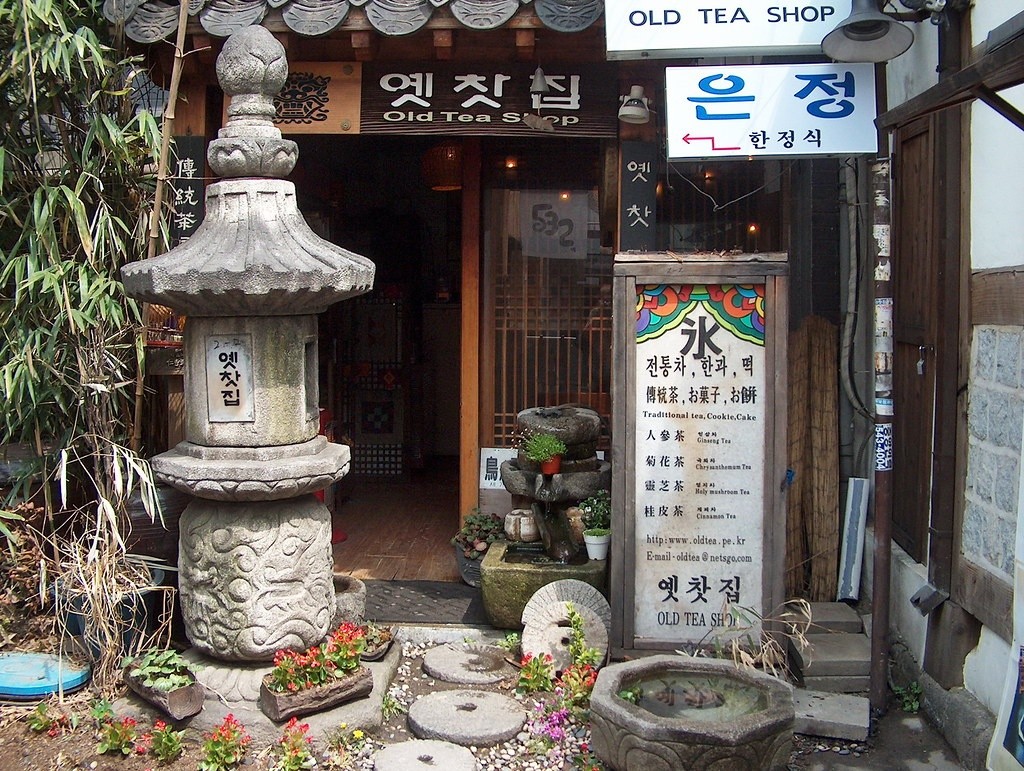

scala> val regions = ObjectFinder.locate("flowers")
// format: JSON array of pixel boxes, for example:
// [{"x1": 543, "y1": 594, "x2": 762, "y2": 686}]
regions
[
  {"x1": 264, "y1": 617, "x2": 369, "y2": 693},
  {"x1": 29, "y1": 700, "x2": 371, "y2": 771},
  {"x1": 518, "y1": 650, "x2": 599, "y2": 764}
]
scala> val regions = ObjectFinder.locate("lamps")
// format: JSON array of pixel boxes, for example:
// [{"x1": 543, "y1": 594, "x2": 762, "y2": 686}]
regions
[
  {"x1": 617, "y1": 83, "x2": 657, "y2": 126},
  {"x1": 819, "y1": 0, "x2": 949, "y2": 65}
]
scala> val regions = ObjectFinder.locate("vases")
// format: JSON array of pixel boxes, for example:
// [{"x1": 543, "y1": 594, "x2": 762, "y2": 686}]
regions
[{"x1": 259, "y1": 665, "x2": 374, "y2": 722}]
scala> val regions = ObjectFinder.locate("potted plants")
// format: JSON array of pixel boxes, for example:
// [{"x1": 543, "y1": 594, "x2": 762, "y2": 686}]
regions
[
  {"x1": 352, "y1": 615, "x2": 400, "y2": 661},
  {"x1": 2, "y1": 1, "x2": 186, "y2": 677},
  {"x1": 122, "y1": 644, "x2": 204, "y2": 719},
  {"x1": 509, "y1": 426, "x2": 567, "y2": 475},
  {"x1": 448, "y1": 507, "x2": 507, "y2": 589},
  {"x1": 577, "y1": 487, "x2": 613, "y2": 561}
]
